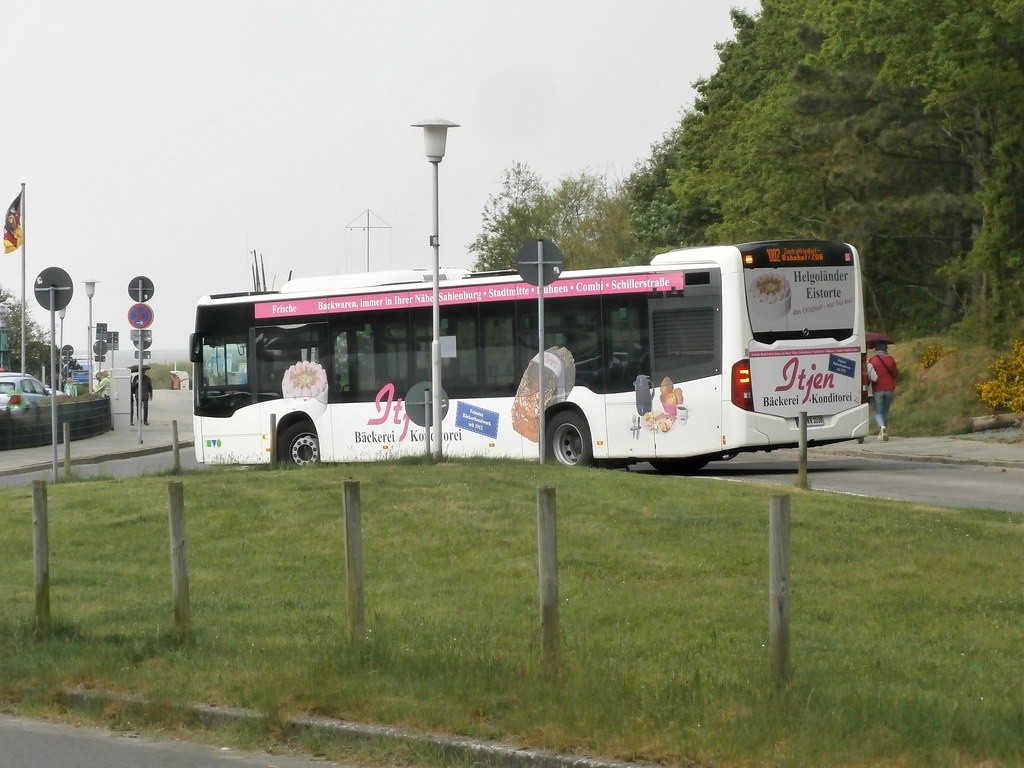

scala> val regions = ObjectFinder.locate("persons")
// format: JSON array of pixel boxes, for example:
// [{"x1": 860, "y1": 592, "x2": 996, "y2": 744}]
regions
[
  {"x1": 63, "y1": 378, "x2": 77, "y2": 397},
  {"x1": 859, "y1": 358, "x2": 877, "y2": 443},
  {"x1": 871, "y1": 344, "x2": 898, "y2": 440},
  {"x1": 132, "y1": 370, "x2": 153, "y2": 426},
  {"x1": 92, "y1": 372, "x2": 111, "y2": 397}
]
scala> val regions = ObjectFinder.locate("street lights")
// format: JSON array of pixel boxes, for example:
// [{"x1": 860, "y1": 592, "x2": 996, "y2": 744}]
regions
[
  {"x1": 57, "y1": 305, "x2": 67, "y2": 390},
  {"x1": 413, "y1": 116, "x2": 461, "y2": 457},
  {"x1": 82, "y1": 279, "x2": 104, "y2": 395}
]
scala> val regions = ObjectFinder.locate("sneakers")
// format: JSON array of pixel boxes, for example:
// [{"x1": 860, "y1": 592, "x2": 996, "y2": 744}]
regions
[{"x1": 877, "y1": 426, "x2": 889, "y2": 441}]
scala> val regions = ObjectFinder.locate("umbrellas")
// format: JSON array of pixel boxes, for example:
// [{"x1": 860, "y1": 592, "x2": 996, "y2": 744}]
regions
[
  {"x1": 128, "y1": 365, "x2": 152, "y2": 373},
  {"x1": 866, "y1": 332, "x2": 895, "y2": 349}
]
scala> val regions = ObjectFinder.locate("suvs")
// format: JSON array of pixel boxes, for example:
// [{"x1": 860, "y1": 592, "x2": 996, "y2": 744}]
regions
[{"x1": 0, "y1": 373, "x2": 48, "y2": 412}]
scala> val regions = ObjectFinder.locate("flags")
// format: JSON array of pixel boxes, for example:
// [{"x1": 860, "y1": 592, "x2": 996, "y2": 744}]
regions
[{"x1": 3, "y1": 191, "x2": 23, "y2": 254}]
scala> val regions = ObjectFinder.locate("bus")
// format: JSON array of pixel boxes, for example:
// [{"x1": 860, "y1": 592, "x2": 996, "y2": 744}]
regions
[{"x1": 187, "y1": 237, "x2": 871, "y2": 474}]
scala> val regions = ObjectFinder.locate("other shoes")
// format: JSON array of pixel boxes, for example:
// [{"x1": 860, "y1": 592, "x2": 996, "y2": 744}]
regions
[
  {"x1": 144, "y1": 419, "x2": 149, "y2": 425},
  {"x1": 131, "y1": 422, "x2": 134, "y2": 425}
]
